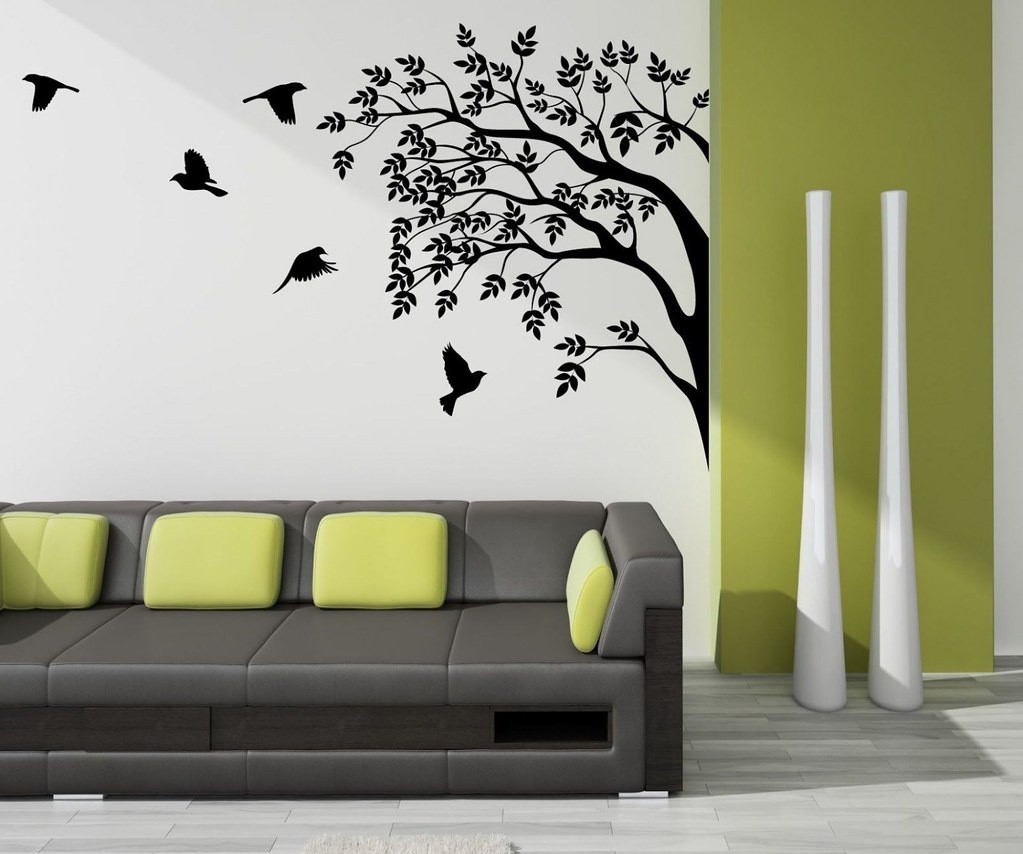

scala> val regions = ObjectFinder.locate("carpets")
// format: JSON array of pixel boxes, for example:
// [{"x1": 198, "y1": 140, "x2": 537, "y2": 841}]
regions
[{"x1": 304, "y1": 832, "x2": 518, "y2": 854}]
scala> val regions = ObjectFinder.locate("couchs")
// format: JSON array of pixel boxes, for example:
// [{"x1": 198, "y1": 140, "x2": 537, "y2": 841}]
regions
[{"x1": 0, "y1": 501, "x2": 686, "y2": 799}]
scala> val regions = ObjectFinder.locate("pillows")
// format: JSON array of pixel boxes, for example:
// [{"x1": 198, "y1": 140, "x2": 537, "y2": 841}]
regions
[
  {"x1": 312, "y1": 511, "x2": 449, "y2": 609},
  {"x1": 143, "y1": 511, "x2": 284, "y2": 609},
  {"x1": 0, "y1": 511, "x2": 109, "y2": 610},
  {"x1": 565, "y1": 529, "x2": 614, "y2": 653}
]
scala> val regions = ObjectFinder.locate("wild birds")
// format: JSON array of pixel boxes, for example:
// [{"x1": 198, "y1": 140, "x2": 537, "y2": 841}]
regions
[
  {"x1": 168, "y1": 149, "x2": 228, "y2": 199},
  {"x1": 243, "y1": 83, "x2": 308, "y2": 124},
  {"x1": 439, "y1": 341, "x2": 487, "y2": 416},
  {"x1": 22, "y1": 73, "x2": 78, "y2": 112},
  {"x1": 272, "y1": 247, "x2": 337, "y2": 294}
]
